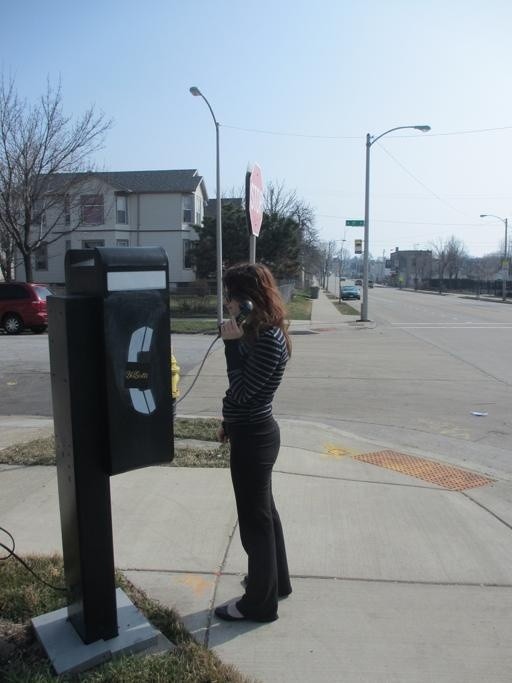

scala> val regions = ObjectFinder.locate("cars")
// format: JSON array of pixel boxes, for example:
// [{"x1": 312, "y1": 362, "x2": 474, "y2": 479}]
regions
[
  {"x1": 339, "y1": 275, "x2": 347, "y2": 281},
  {"x1": 341, "y1": 285, "x2": 362, "y2": 300},
  {"x1": 0, "y1": 281, "x2": 57, "y2": 336},
  {"x1": 355, "y1": 280, "x2": 363, "y2": 285}
]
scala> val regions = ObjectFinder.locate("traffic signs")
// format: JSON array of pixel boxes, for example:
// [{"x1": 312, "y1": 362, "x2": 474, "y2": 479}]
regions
[{"x1": 345, "y1": 219, "x2": 365, "y2": 226}]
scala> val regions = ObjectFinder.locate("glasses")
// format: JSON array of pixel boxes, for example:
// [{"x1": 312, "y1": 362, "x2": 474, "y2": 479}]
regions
[{"x1": 224, "y1": 290, "x2": 234, "y2": 303}]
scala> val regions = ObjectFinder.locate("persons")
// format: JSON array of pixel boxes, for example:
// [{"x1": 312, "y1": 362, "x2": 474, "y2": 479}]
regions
[{"x1": 216, "y1": 265, "x2": 293, "y2": 621}]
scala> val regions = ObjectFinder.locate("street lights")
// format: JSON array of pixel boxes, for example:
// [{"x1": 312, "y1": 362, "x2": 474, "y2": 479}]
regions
[
  {"x1": 359, "y1": 125, "x2": 431, "y2": 323},
  {"x1": 480, "y1": 213, "x2": 508, "y2": 301},
  {"x1": 189, "y1": 84, "x2": 225, "y2": 341},
  {"x1": 326, "y1": 239, "x2": 348, "y2": 291}
]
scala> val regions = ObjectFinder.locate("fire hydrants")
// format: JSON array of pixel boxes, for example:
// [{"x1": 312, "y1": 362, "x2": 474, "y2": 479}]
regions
[{"x1": 170, "y1": 349, "x2": 181, "y2": 416}]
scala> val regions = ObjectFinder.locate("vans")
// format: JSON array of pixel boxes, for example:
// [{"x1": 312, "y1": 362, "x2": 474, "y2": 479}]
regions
[{"x1": 367, "y1": 279, "x2": 374, "y2": 288}]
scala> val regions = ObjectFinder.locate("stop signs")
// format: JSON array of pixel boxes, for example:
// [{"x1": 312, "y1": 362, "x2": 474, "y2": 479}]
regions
[{"x1": 248, "y1": 161, "x2": 266, "y2": 237}]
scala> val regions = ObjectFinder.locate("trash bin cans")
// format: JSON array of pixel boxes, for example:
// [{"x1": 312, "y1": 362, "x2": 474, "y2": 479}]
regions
[{"x1": 310, "y1": 287, "x2": 319, "y2": 299}]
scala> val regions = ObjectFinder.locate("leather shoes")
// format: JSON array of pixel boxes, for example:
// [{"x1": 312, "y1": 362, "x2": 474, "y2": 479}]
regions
[
  {"x1": 214, "y1": 602, "x2": 277, "y2": 622},
  {"x1": 243, "y1": 567, "x2": 292, "y2": 598}
]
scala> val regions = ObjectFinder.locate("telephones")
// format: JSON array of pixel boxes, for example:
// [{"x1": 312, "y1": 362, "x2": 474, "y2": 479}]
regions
[{"x1": 218, "y1": 300, "x2": 253, "y2": 335}]
